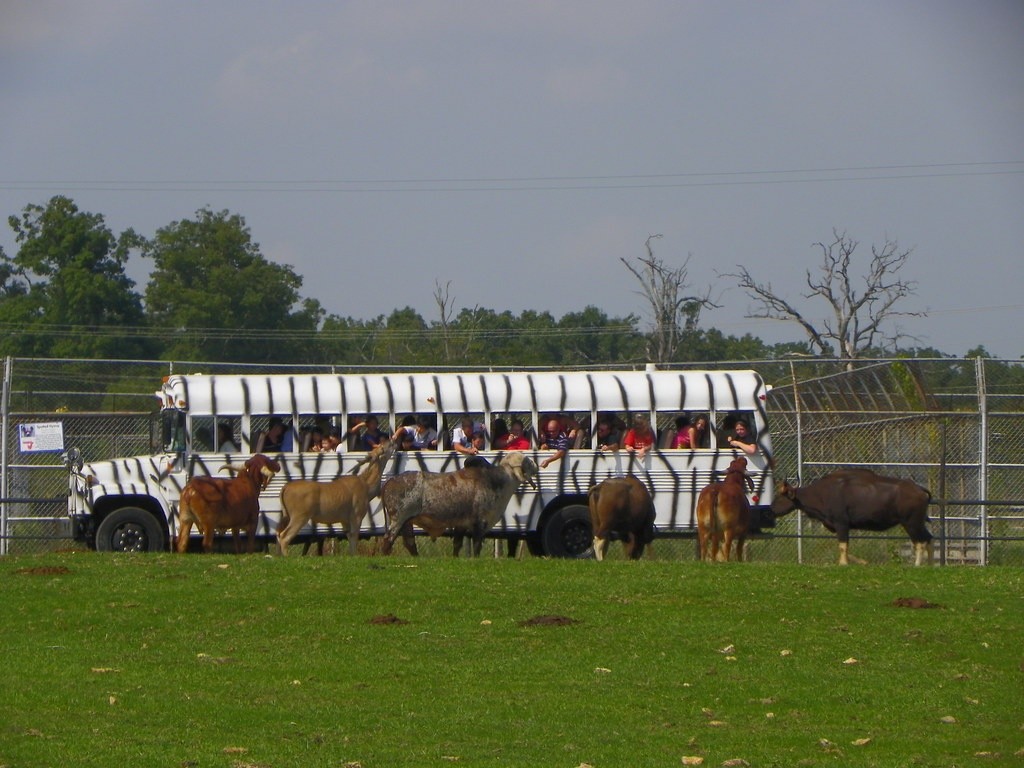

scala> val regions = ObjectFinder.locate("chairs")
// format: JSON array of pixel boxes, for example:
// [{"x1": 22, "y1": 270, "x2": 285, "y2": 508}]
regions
[
  {"x1": 251, "y1": 430, "x2": 266, "y2": 453},
  {"x1": 573, "y1": 429, "x2": 583, "y2": 449},
  {"x1": 657, "y1": 428, "x2": 675, "y2": 448},
  {"x1": 349, "y1": 431, "x2": 361, "y2": 451},
  {"x1": 618, "y1": 429, "x2": 630, "y2": 449},
  {"x1": 300, "y1": 430, "x2": 312, "y2": 452}
]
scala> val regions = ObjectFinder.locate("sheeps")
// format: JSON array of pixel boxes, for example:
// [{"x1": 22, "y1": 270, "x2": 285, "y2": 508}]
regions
[{"x1": 277, "y1": 438, "x2": 398, "y2": 556}]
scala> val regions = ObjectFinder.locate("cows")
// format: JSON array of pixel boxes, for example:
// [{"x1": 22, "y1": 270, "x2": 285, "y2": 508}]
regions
[
  {"x1": 588, "y1": 475, "x2": 656, "y2": 564},
  {"x1": 175, "y1": 453, "x2": 281, "y2": 556},
  {"x1": 697, "y1": 455, "x2": 758, "y2": 567},
  {"x1": 770, "y1": 468, "x2": 934, "y2": 568},
  {"x1": 382, "y1": 452, "x2": 539, "y2": 557}
]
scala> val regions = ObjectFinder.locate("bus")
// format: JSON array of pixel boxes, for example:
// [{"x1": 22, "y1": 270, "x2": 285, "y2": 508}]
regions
[{"x1": 58, "y1": 363, "x2": 778, "y2": 560}]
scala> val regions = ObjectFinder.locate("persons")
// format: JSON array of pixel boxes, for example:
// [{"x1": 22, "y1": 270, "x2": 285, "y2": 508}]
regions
[{"x1": 196, "y1": 412, "x2": 758, "y2": 468}]
